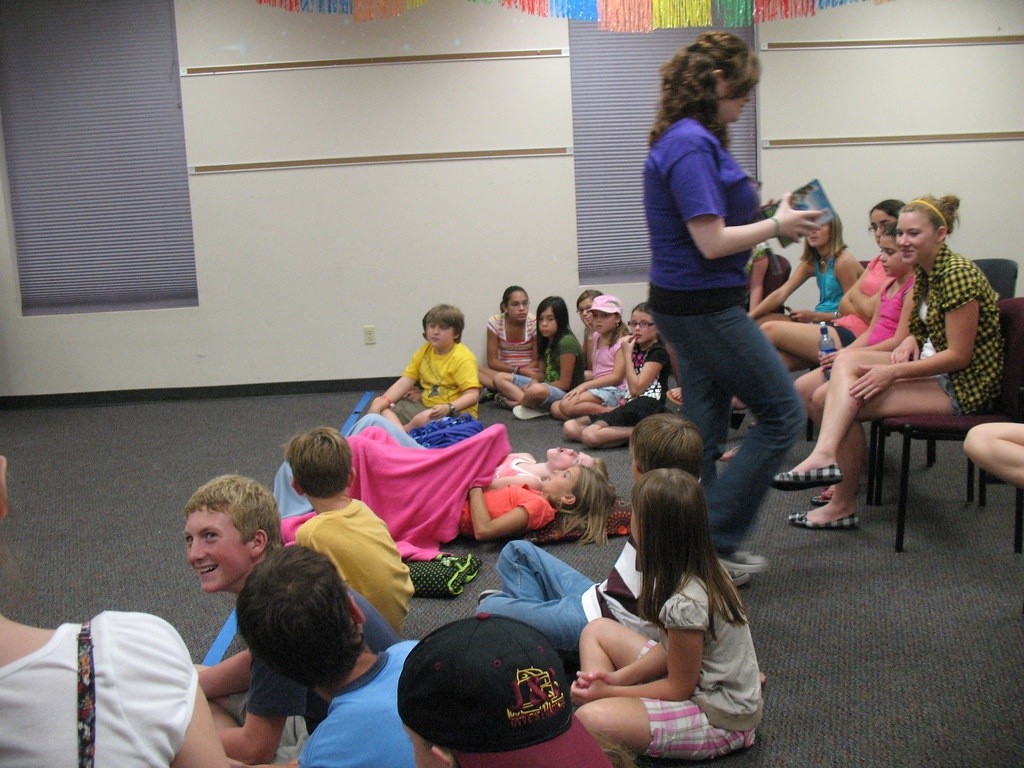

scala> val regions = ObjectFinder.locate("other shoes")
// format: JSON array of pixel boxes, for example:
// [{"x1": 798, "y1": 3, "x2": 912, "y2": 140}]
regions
[
  {"x1": 769, "y1": 463, "x2": 841, "y2": 490},
  {"x1": 716, "y1": 558, "x2": 755, "y2": 586},
  {"x1": 731, "y1": 550, "x2": 769, "y2": 573},
  {"x1": 788, "y1": 512, "x2": 860, "y2": 529},
  {"x1": 494, "y1": 392, "x2": 513, "y2": 405}
]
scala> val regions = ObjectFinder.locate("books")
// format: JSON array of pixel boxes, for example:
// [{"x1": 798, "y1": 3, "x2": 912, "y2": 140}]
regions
[{"x1": 759, "y1": 179, "x2": 838, "y2": 248}]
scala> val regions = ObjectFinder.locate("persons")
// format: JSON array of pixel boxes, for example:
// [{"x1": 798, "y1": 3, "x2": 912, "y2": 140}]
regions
[
  {"x1": 549, "y1": 294, "x2": 630, "y2": 419},
  {"x1": 745, "y1": 210, "x2": 866, "y2": 330},
  {"x1": 576, "y1": 290, "x2": 604, "y2": 382},
  {"x1": 478, "y1": 285, "x2": 540, "y2": 392},
  {"x1": 963, "y1": 422, "x2": 1024, "y2": 489},
  {"x1": 235, "y1": 545, "x2": 422, "y2": 768},
  {"x1": 793, "y1": 222, "x2": 917, "y2": 506},
  {"x1": 643, "y1": 32, "x2": 825, "y2": 587},
  {"x1": 475, "y1": 412, "x2": 703, "y2": 666},
  {"x1": 666, "y1": 199, "x2": 907, "y2": 410},
  {"x1": 280, "y1": 426, "x2": 415, "y2": 633},
  {"x1": 367, "y1": 304, "x2": 483, "y2": 434},
  {"x1": 486, "y1": 446, "x2": 608, "y2": 491},
  {"x1": 181, "y1": 474, "x2": 402, "y2": 766},
  {"x1": 492, "y1": 296, "x2": 585, "y2": 419},
  {"x1": 742, "y1": 241, "x2": 769, "y2": 313},
  {"x1": 562, "y1": 301, "x2": 671, "y2": 449},
  {"x1": 0, "y1": 455, "x2": 231, "y2": 768},
  {"x1": 397, "y1": 612, "x2": 613, "y2": 768},
  {"x1": 570, "y1": 468, "x2": 763, "y2": 761},
  {"x1": 458, "y1": 464, "x2": 617, "y2": 549},
  {"x1": 770, "y1": 195, "x2": 1005, "y2": 530}
]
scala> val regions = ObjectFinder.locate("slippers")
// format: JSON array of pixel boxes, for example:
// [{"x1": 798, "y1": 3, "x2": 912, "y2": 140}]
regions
[
  {"x1": 665, "y1": 386, "x2": 685, "y2": 406},
  {"x1": 811, "y1": 490, "x2": 836, "y2": 504}
]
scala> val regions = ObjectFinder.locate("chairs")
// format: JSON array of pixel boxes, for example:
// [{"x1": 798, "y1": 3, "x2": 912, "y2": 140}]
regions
[{"x1": 808, "y1": 259, "x2": 1024, "y2": 557}]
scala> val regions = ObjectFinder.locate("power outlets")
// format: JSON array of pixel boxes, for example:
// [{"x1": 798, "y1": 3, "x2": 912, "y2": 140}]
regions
[{"x1": 363, "y1": 325, "x2": 376, "y2": 345}]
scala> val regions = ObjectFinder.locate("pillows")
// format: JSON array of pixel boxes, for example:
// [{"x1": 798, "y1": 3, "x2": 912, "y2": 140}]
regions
[{"x1": 523, "y1": 493, "x2": 631, "y2": 542}]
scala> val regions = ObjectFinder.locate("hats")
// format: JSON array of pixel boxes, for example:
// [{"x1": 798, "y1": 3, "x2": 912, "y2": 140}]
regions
[
  {"x1": 396, "y1": 612, "x2": 611, "y2": 768},
  {"x1": 586, "y1": 295, "x2": 622, "y2": 316}
]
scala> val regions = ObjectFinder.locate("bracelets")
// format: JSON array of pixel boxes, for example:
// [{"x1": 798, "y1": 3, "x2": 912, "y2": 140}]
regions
[
  {"x1": 380, "y1": 395, "x2": 395, "y2": 408},
  {"x1": 834, "y1": 311, "x2": 839, "y2": 320},
  {"x1": 512, "y1": 367, "x2": 520, "y2": 374},
  {"x1": 770, "y1": 217, "x2": 779, "y2": 239},
  {"x1": 447, "y1": 403, "x2": 456, "y2": 414},
  {"x1": 466, "y1": 485, "x2": 484, "y2": 498}
]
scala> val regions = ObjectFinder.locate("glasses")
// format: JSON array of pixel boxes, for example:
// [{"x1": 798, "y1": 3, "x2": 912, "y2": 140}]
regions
[
  {"x1": 869, "y1": 223, "x2": 889, "y2": 233},
  {"x1": 628, "y1": 320, "x2": 656, "y2": 331},
  {"x1": 575, "y1": 308, "x2": 589, "y2": 315}
]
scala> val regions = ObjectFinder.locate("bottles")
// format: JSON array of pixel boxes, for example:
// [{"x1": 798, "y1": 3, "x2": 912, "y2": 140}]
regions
[{"x1": 818, "y1": 326, "x2": 837, "y2": 381}]
